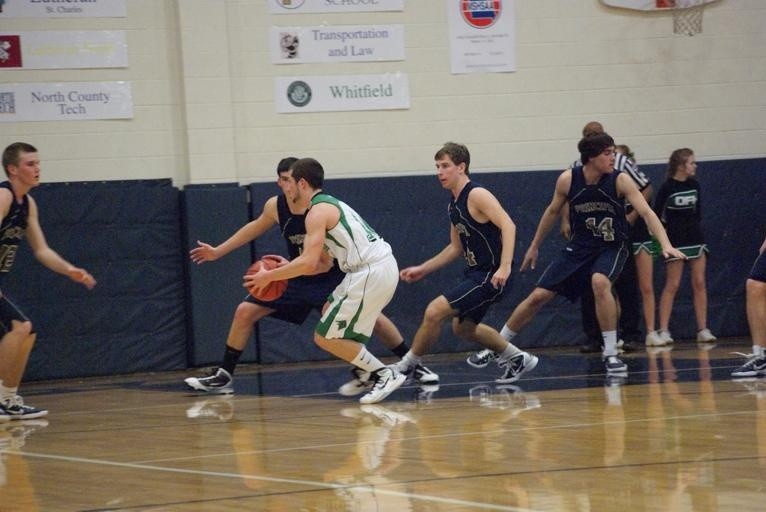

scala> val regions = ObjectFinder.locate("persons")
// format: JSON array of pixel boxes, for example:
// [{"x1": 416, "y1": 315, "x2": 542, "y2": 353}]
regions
[
  {"x1": 0, "y1": 419, "x2": 49, "y2": 484},
  {"x1": 731, "y1": 240, "x2": 766, "y2": 377},
  {"x1": 184, "y1": 158, "x2": 440, "y2": 396},
  {"x1": 385, "y1": 143, "x2": 539, "y2": 385},
  {"x1": 466, "y1": 132, "x2": 689, "y2": 372},
  {"x1": 560, "y1": 118, "x2": 717, "y2": 355},
  {"x1": 240, "y1": 157, "x2": 407, "y2": 405},
  {"x1": 0, "y1": 143, "x2": 97, "y2": 423}
]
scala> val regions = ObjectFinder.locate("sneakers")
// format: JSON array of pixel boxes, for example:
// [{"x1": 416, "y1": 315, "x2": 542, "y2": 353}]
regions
[
  {"x1": 494, "y1": 350, "x2": 539, "y2": 385},
  {"x1": 1, "y1": 397, "x2": 50, "y2": 421},
  {"x1": 731, "y1": 348, "x2": 766, "y2": 377},
  {"x1": 646, "y1": 331, "x2": 674, "y2": 347},
  {"x1": 602, "y1": 350, "x2": 626, "y2": 372},
  {"x1": 184, "y1": 368, "x2": 235, "y2": 394},
  {"x1": 697, "y1": 329, "x2": 716, "y2": 342},
  {"x1": 339, "y1": 362, "x2": 440, "y2": 403},
  {"x1": 467, "y1": 349, "x2": 499, "y2": 367}
]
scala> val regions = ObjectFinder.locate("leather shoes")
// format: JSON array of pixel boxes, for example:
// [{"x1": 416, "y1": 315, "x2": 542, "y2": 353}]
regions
[
  {"x1": 580, "y1": 339, "x2": 599, "y2": 352},
  {"x1": 622, "y1": 340, "x2": 640, "y2": 350}
]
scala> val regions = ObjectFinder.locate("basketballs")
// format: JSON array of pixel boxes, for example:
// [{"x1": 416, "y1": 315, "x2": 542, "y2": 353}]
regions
[{"x1": 246, "y1": 259, "x2": 286, "y2": 301}]
